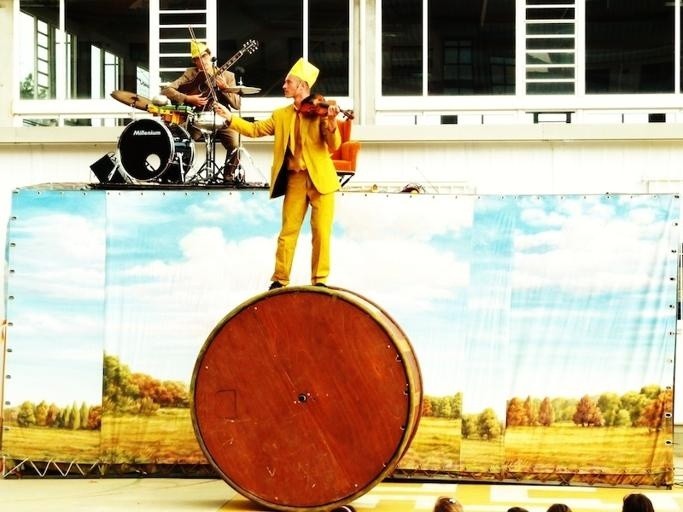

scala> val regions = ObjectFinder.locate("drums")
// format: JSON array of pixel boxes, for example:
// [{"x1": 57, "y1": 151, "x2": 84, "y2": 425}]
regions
[
  {"x1": 115, "y1": 118, "x2": 196, "y2": 183},
  {"x1": 190, "y1": 112, "x2": 226, "y2": 133},
  {"x1": 151, "y1": 107, "x2": 188, "y2": 126}
]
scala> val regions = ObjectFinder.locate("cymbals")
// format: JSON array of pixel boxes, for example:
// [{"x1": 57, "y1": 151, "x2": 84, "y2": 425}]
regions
[
  {"x1": 222, "y1": 85, "x2": 261, "y2": 96},
  {"x1": 110, "y1": 90, "x2": 153, "y2": 111}
]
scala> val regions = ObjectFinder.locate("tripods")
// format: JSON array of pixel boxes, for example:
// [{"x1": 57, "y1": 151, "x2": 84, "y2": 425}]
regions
[
  {"x1": 207, "y1": 94, "x2": 270, "y2": 188},
  {"x1": 183, "y1": 63, "x2": 225, "y2": 186}
]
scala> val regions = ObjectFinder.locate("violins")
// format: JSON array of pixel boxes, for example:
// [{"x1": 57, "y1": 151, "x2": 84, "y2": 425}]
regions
[{"x1": 299, "y1": 94, "x2": 354, "y2": 122}]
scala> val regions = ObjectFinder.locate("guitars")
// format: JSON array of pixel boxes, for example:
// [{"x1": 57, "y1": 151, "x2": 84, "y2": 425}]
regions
[{"x1": 178, "y1": 39, "x2": 259, "y2": 102}]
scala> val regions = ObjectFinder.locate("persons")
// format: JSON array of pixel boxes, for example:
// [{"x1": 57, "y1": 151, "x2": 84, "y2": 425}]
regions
[
  {"x1": 213, "y1": 56, "x2": 342, "y2": 291},
  {"x1": 433, "y1": 496, "x2": 465, "y2": 512},
  {"x1": 506, "y1": 507, "x2": 528, "y2": 511},
  {"x1": 333, "y1": 504, "x2": 357, "y2": 512},
  {"x1": 159, "y1": 41, "x2": 242, "y2": 182},
  {"x1": 622, "y1": 493, "x2": 655, "y2": 511},
  {"x1": 549, "y1": 503, "x2": 572, "y2": 511}
]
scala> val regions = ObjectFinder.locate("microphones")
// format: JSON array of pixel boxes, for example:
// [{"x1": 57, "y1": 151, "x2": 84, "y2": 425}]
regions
[
  {"x1": 235, "y1": 66, "x2": 244, "y2": 74},
  {"x1": 209, "y1": 56, "x2": 217, "y2": 63}
]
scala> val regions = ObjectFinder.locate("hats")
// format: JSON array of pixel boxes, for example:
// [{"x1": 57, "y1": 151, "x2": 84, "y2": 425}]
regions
[
  {"x1": 287, "y1": 58, "x2": 322, "y2": 89},
  {"x1": 191, "y1": 40, "x2": 204, "y2": 58}
]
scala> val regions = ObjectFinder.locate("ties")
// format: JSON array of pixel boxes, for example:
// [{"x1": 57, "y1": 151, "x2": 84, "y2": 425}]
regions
[{"x1": 293, "y1": 104, "x2": 302, "y2": 158}]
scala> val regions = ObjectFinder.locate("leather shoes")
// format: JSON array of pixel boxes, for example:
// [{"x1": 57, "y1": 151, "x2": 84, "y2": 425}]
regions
[{"x1": 268, "y1": 281, "x2": 284, "y2": 290}]
